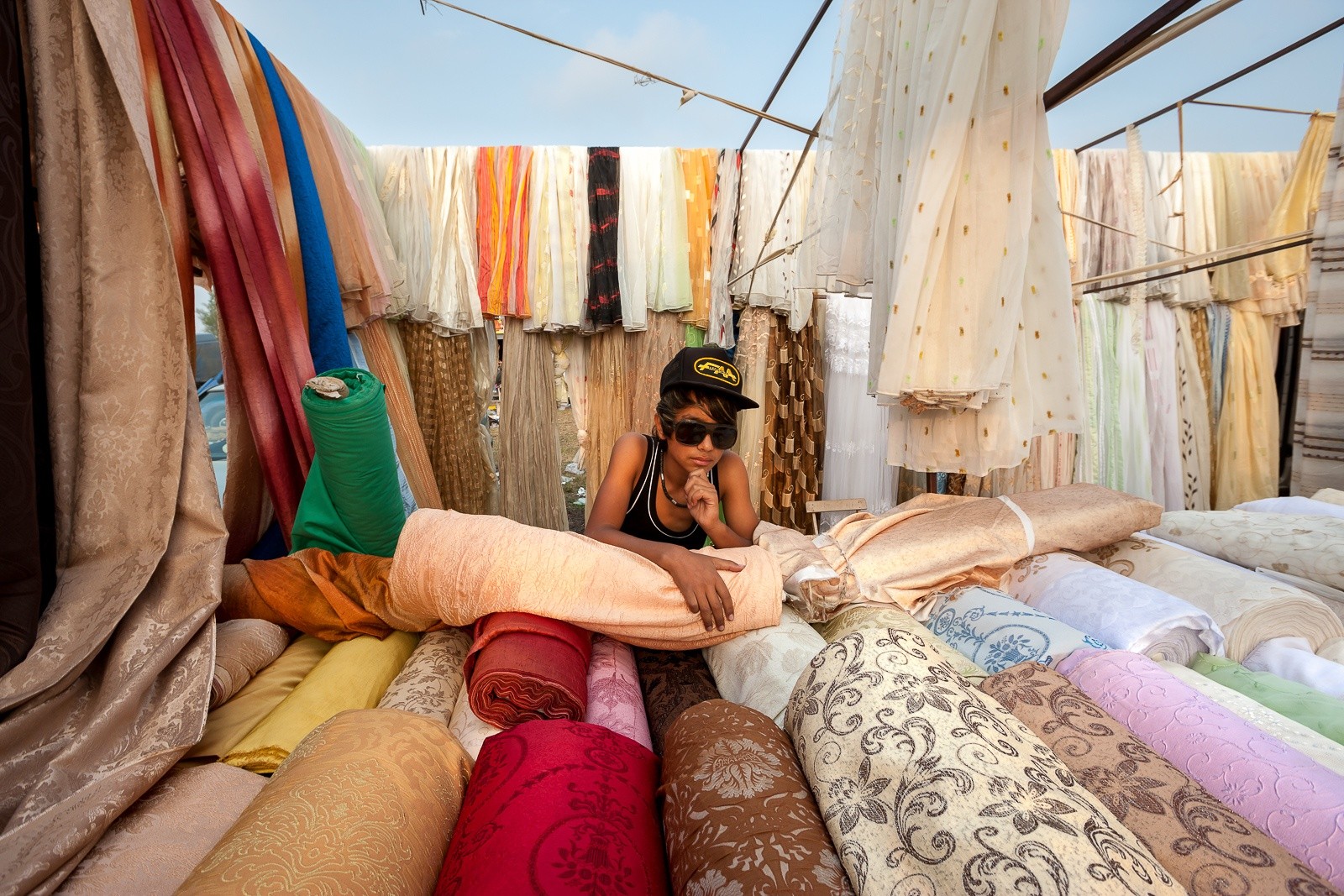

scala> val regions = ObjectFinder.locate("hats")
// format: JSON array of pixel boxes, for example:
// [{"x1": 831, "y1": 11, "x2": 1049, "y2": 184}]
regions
[{"x1": 660, "y1": 347, "x2": 759, "y2": 410}]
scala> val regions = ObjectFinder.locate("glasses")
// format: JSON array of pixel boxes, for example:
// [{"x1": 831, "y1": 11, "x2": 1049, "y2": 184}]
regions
[{"x1": 662, "y1": 414, "x2": 737, "y2": 450}]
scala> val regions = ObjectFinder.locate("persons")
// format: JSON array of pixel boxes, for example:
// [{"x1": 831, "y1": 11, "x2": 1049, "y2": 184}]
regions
[{"x1": 583, "y1": 348, "x2": 760, "y2": 632}]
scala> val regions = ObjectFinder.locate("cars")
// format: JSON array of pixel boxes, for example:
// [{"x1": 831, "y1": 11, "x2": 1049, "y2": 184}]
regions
[
  {"x1": 197, "y1": 368, "x2": 228, "y2": 510},
  {"x1": 194, "y1": 331, "x2": 223, "y2": 388}
]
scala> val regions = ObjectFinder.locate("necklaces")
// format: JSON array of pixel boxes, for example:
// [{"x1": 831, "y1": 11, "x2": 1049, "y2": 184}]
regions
[{"x1": 660, "y1": 448, "x2": 710, "y2": 508}]
